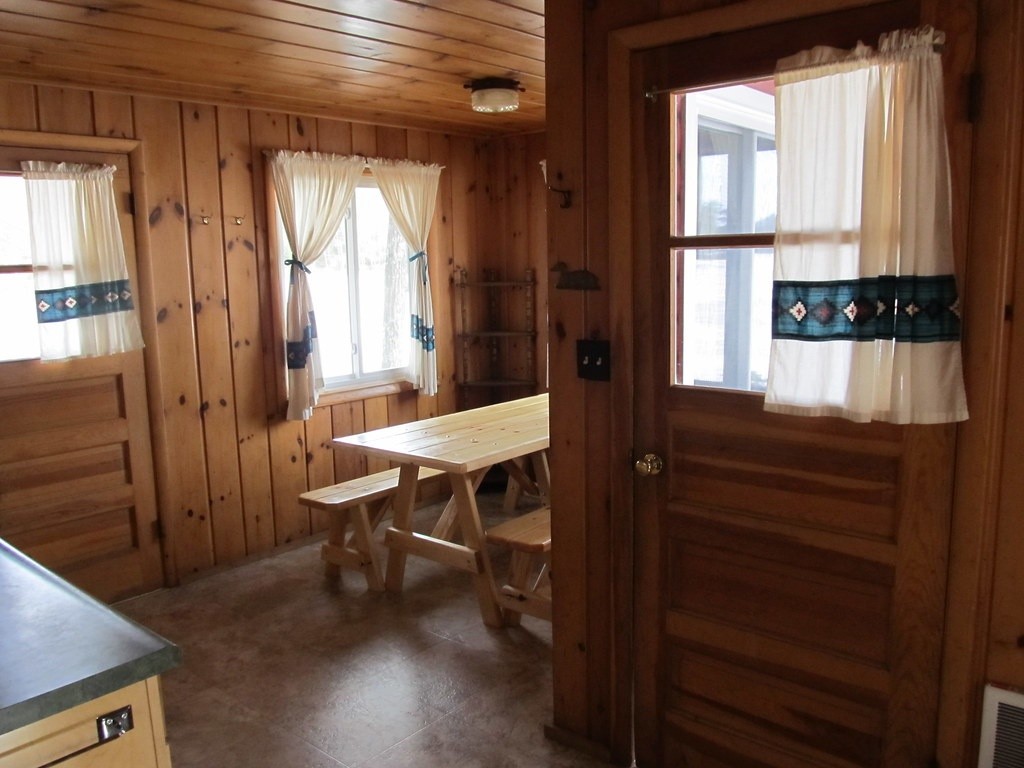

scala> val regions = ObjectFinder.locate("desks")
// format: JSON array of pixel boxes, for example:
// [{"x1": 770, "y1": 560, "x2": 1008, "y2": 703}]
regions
[{"x1": 333, "y1": 392, "x2": 549, "y2": 628}]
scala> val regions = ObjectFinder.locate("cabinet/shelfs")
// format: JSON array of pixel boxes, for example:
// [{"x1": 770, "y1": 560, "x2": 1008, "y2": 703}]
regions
[
  {"x1": 450, "y1": 269, "x2": 537, "y2": 484},
  {"x1": 0, "y1": 540, "x2": 182, "y2": 768}
]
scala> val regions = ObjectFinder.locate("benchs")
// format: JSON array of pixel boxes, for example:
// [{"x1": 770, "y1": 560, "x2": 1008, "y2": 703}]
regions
[
  {"x1": 484, "y1": 505, "x2": 551, "y2": 627},
  {"x1": 298, "y1": 465, "x2": 449, "y2": 595}
]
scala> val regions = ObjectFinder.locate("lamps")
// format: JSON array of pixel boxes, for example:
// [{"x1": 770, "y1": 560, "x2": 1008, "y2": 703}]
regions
[{"x1": 463, "y1": 76, "x2": 526, "y2": 114}]
